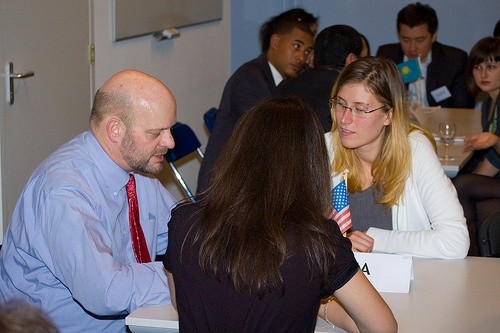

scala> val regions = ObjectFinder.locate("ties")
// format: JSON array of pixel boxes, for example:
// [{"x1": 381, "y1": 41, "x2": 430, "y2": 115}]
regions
[{"x1": 125, "y1": 174, "x2": 154, "y2": 262}]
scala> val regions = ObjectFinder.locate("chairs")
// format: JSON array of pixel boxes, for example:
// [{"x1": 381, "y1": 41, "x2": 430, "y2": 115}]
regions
[
  {"x1": 478, "y1": 209, "x2": 500, "y2": 258},
  {"x1": 203, "y1": 107, "x2": 218, "y2": 133},
  {"x1": 165, "y1": 123, "x2": 205, "y2": 203}
]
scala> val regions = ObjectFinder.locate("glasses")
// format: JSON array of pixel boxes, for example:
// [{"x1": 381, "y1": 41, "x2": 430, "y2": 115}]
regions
[{"x1": 330, "y1": 97, "x2": 389, "y2": 118}]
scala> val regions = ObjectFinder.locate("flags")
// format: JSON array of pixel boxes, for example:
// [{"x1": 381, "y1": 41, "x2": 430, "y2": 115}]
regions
[
  {"x1": 324, "y1": 168, "x2": 352, "y2": 233},
  {"x1": 395, "y1": 58, "x2": 425, "y2": 83}
]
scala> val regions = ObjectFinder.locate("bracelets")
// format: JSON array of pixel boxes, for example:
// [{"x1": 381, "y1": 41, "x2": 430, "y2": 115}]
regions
[{"x1": 324, "y1": 299, "x2": 334, "y2": 330}]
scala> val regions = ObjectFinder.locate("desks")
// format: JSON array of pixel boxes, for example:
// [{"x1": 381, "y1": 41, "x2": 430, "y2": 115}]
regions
[
  {"x1": 125, "y1": 254, "x2": 500, "y2": 333},
  {"x1": 409, "y1": 106, "x2": 483, "y2": 176}
]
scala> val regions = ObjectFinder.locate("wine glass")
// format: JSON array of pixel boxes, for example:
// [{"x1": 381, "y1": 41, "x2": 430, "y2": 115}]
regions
[{"x1": 439, "y1": 122, "x2": 456, "y2": 161}]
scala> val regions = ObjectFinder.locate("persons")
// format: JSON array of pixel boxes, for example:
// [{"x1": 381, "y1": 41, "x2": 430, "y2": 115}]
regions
[
  {"x1": 1, "y1": 69, "x2": 179, "y2": 333},
  {"x1": 279, "y1": 2, "x2": 500, "y2": 134},
  {"x1": 195, "y1": 16, "x2": 314, "y2": 209},
  {"x1": 0, "y1": 298, "x2": 59, "y2": 332},
  {"x1": 162, "y1": 93, "x2": 399, "y2": 333},
  {"x1": 449, "y1": 36, "x2": 500, "y2": 257},
  {"x1": 320, "y1": 55, "x2": 471, "y2": 261}
]
